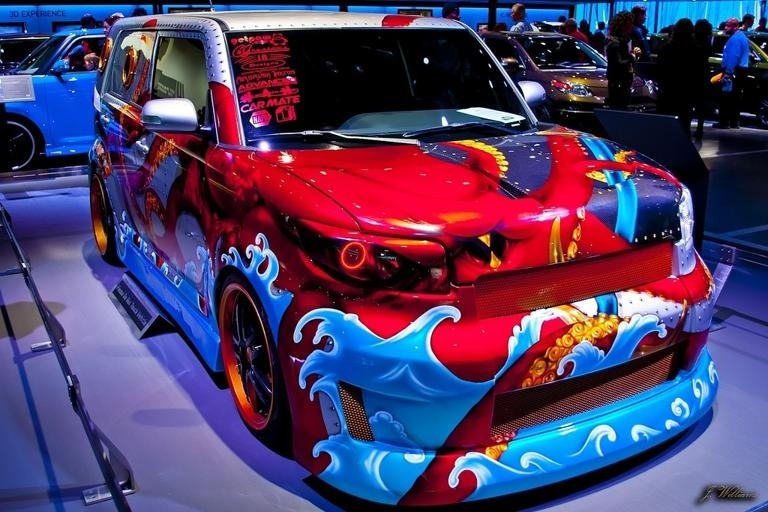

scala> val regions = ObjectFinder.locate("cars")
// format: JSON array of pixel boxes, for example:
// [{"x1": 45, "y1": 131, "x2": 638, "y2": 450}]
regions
[{"x1": 0, "y1": 10, "x2": 768, "y2": 506}]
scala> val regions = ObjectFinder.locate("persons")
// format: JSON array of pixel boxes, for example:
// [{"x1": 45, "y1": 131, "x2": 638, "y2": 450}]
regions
[
  {"x1": 82, "y1": 54, "x2": 100, "y2": 71},
  {"x1": 80, "y1": 13, "x2": 96, "y2": 29},
  {"x1": 103, "y1": 15, "x2": 119, "y2": 36},
  {"x1": 131, "y1": 8, "x2": 148, "y2": 17},
  {"x1": 442, "y1": 0, "x2": 768, "y2": 135},
  {"x1": 64, "y1": 39, "x2": 100, "y2": 71}
]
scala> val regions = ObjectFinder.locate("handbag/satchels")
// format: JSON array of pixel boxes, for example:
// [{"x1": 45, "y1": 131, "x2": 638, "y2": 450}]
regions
[{"x1": 720, "y1": 78, "x2": 732, "y2": 92}]
